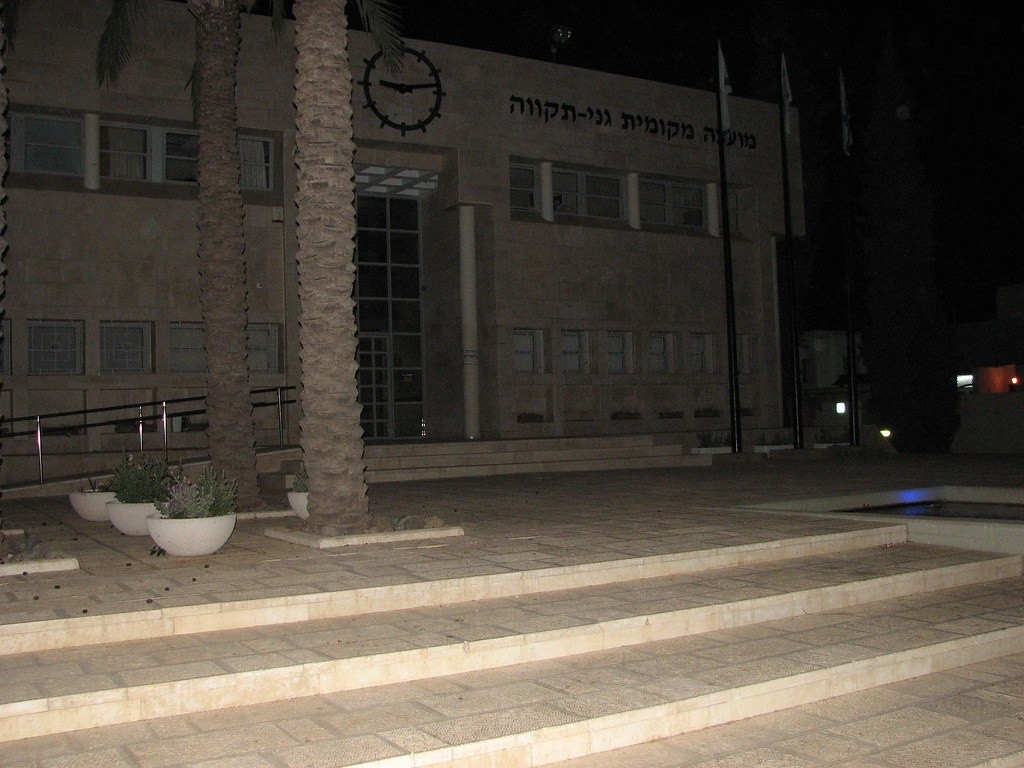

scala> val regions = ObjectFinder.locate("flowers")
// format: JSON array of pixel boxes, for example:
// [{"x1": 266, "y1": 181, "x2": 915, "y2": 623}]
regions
[{"x1": 153, "y1": 465, "x2": 240, "y2": 518}]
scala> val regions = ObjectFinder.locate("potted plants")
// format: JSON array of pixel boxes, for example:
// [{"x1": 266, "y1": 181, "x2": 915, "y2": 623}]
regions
[
  {"x1": 286, "y1": 460, "x2": 310, "y2": 521},
  {"x1": 68, "y1": 477, "x2": 124, "y2": 522},
  {"x1": 105, "y1": 446, "x2": 175, "y2": 537}
]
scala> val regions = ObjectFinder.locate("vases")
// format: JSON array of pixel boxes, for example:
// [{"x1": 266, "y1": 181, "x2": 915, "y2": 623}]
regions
[{"x1": 145, "y1": 512, "x2": 237, "y2": 556}]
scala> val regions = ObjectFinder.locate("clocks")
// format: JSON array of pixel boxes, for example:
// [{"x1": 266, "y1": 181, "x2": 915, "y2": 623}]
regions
[{"x1": 357, "y1": 40, "x2": 446, "y2": 137}]
